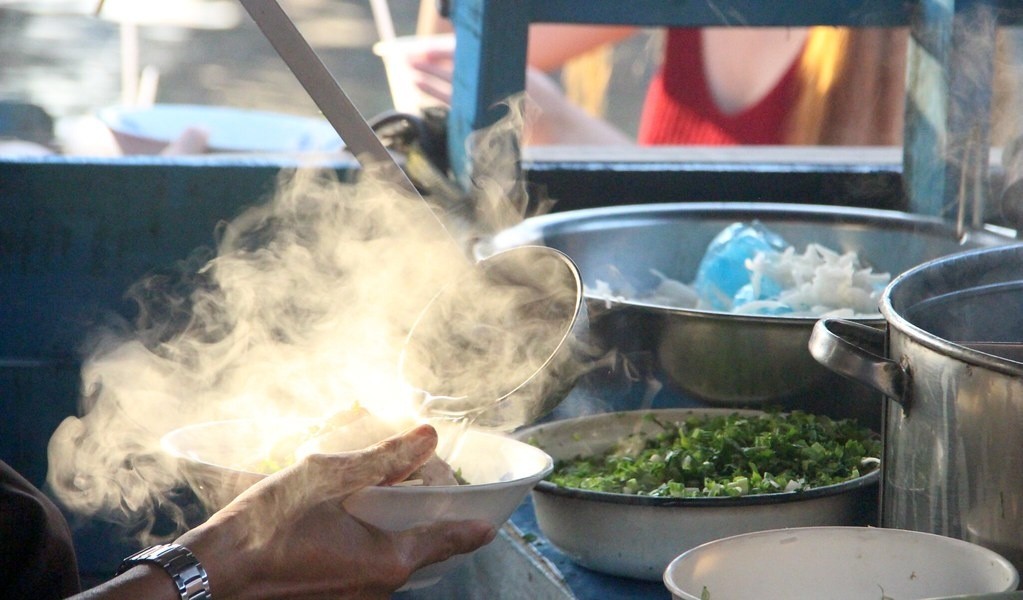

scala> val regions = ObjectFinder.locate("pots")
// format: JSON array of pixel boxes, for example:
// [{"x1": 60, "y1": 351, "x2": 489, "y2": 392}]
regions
[{"x1": 807, "y1": 244, "x2": 1023, "y2": 593}]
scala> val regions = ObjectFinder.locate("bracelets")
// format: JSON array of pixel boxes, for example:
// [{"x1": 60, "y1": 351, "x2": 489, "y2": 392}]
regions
[{"x1": 114, "y1": 541, "x2": 213, "y2": 600}]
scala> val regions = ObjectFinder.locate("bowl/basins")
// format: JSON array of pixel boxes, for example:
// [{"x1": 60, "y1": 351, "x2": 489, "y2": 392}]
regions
[
  {"x1": 110, "y1": 105, "x2": 344, "y2": 153},
  {"x1": 483, "y1": 200, "x2": 1018, "y2": 420},
  {"x1": 165, "y1": 421, "x2": 554, "y2": 591},
  {"x1": 662, "y1": 525, "x2": 1019, "y2": 600},
  {"x1": 507, "y1": 407, "x2": 879, "y2": 582}
]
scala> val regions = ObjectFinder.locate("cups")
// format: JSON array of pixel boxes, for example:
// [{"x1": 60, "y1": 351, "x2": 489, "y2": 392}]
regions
[{"x1": 371, "y1": 35, "x2": 454, "y2": 113}]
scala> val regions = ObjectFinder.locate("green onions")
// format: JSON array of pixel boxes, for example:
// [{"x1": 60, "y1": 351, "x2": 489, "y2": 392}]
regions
[
  {"x1": 452, "y1": 469, "x2": 543, "y2": 546},
  {"x1": 531, "y1": 408, "x2": 880, "y2": 499}
]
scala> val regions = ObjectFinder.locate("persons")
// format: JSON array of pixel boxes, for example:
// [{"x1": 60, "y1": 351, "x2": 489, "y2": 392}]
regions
[
  {"x1": 0, "y1": 423, "x2": 496, "y2": 600},
  {"x1": 374, "y1": 0, "x2": 907, "y2": 151}
]
